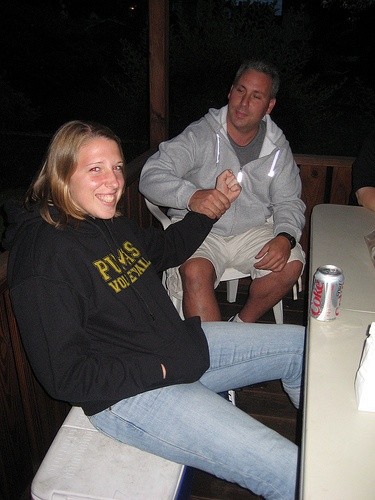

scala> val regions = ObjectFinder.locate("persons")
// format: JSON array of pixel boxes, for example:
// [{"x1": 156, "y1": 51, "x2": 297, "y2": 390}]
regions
[
  {"x1": 138, "y1": 57, "x2": 306, "y2": 322},
  {"x1": 7, "y1": 120, "x2": 306, "y2": 499},
  {"x1": 350, "y1": 131, "x2": 374, "y2": 211}
]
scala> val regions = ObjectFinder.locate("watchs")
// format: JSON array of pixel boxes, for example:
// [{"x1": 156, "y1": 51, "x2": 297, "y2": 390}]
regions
[{"x1": 277, "y1": 232, "x2": 296, "y2": 249}]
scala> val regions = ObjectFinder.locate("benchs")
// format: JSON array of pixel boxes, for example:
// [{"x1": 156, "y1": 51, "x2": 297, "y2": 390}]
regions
[
  {"x1": 30, "y1": 403, "x2": 188, "y2": 500},
  {"x1": 238, "y1": 153, "x2": 357, "y2": 292}
]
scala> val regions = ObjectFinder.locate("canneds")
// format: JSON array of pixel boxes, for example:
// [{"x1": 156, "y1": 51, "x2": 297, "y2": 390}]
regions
[{"x1": 309, "y1": 265, "x2": 344, "y2": 322}]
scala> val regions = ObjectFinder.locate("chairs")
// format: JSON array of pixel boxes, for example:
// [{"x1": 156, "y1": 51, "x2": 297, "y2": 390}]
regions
[{"x1": 141, "y1": 188, "x2": 284, "y2": 327}]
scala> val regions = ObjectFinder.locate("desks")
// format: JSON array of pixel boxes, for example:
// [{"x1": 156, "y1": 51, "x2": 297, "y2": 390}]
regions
[{"x1": 299, "y1": 203, "x2": 375, "y2": 500}]
